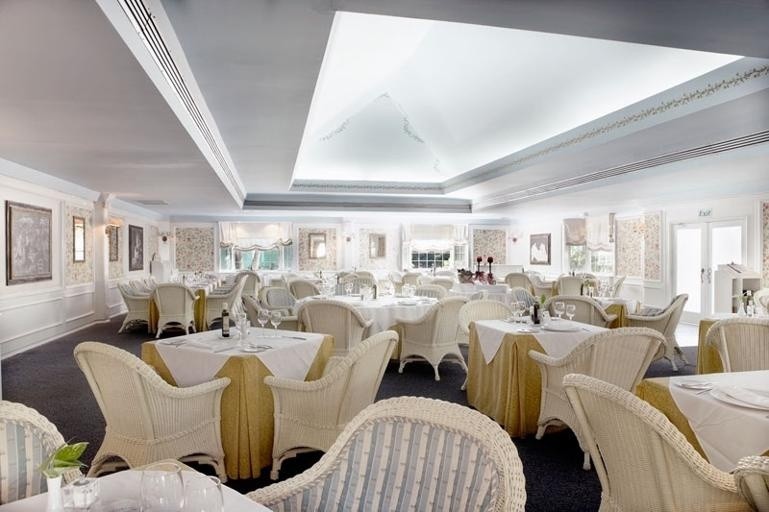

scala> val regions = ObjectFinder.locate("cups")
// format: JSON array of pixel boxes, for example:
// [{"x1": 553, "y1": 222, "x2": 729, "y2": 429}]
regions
[{"x1": 140, "y1": 462, "x2": 223, "y2": 512}]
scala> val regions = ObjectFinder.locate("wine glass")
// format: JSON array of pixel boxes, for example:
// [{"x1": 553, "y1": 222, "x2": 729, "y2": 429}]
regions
[
  {"x1": 554, "y1": 302, "x2": 575, "y2": 320},
  {"x1": 510, "y1": 301, "x2": 526, "y2": 323},
  {"x1": 318, "y1": 283, "x2": 326, "y2": 299},
  {"x1": 345, "y1": 283, "x2": 353, "y2": 298},
  {"x1": 236, "y1": 309, "x2": 281, "y2": 341}
]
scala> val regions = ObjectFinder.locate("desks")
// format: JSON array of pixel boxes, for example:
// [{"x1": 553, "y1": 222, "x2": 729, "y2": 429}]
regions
[
  {"x1": 634, "y1": 370, "x2": 769, "y2": 472},
  {"x1": 705, "y1": 318, "x2": 764, "y2": 368},
  {"x1": 467, "y1": 316, "x2": 612, "y2": 432},
  {"x1": 140, "y1": 326, "x2": 333, "y2": 481},
  {"x1": 1, "y1": 458, "x2": 271, "y2": 512}
]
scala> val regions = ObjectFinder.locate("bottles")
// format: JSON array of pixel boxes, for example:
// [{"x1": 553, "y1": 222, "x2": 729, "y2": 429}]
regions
[
  {"x1": 747, "y1": 290, "x2": 753, "y2": 318},
  {"x1": 534, "y1": 296, "x2": 540, "y2": 324},
  {"x1": 222, "y1": 303, "x2": 230, "y2": 337},
  {"x1": 373, "y1": 282, "x2": 377, "y2": 300}
]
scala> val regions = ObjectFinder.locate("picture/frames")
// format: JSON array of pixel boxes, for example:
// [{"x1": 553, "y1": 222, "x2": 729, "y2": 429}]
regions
[
  {"x1": 369, "y1": 232, "x2": 386, "y2": 259},
  {"x1": 529, "y1": 233, "x2": 551, "y2": 266},
  {"x1": 308, "y1": 232, "x2": 327, "y2": 260},
  {"x1": 3, "y1": 199, "x2": 144, "y2": 286}
]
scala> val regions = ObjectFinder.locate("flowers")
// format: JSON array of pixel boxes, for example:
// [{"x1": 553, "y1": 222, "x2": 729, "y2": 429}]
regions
[
  {"x1": 487, "y1": 256, "x2": 493, "y2": 274},
  {"x1": 477, "y1": 256, "x2": 483, "y2": 272}
]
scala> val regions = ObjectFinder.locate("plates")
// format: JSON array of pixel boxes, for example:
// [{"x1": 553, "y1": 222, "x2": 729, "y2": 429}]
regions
[
  {"x1": 710, "y1": 390, "x2": 769, "y2": 411},
  {"x1": 241, "y1": 346, "x2": 266, "y2": 352}
]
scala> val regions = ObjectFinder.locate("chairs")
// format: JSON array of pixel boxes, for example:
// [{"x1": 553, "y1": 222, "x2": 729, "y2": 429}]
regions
[
  {"x1": 263, "y1": 331, "x2": 397, "y2": 479},
  {"x1": 115, "y1": 268, "x2": 690, "y2": 335},
  {"x1": 292, "y1": 299, "x2": 376, "y2": 359},
  {"x1": 73, "y1": 340, "x2": 233, "y2": 486},
  {"x1": 0, "y1": 398, "x2": 71, "y2": 498},
  {"x1": 696, "y1": 318, "x2": 768, "y2": 373},
  {"x1": 535, "y1": 327, "x2": 662, "y2": 471},
  {"x1": 401, "y1": 295, "x2": 469, "y2": 380},
  {"x1": 561, "y1": 375, "x2": 751, "y2": 511},
  {"x1": 246, "y1": 395, "x2": 527, "y2": 511},
  {"x1": 737, "y1": 447, "x2": 764, "y2": 505}
]
